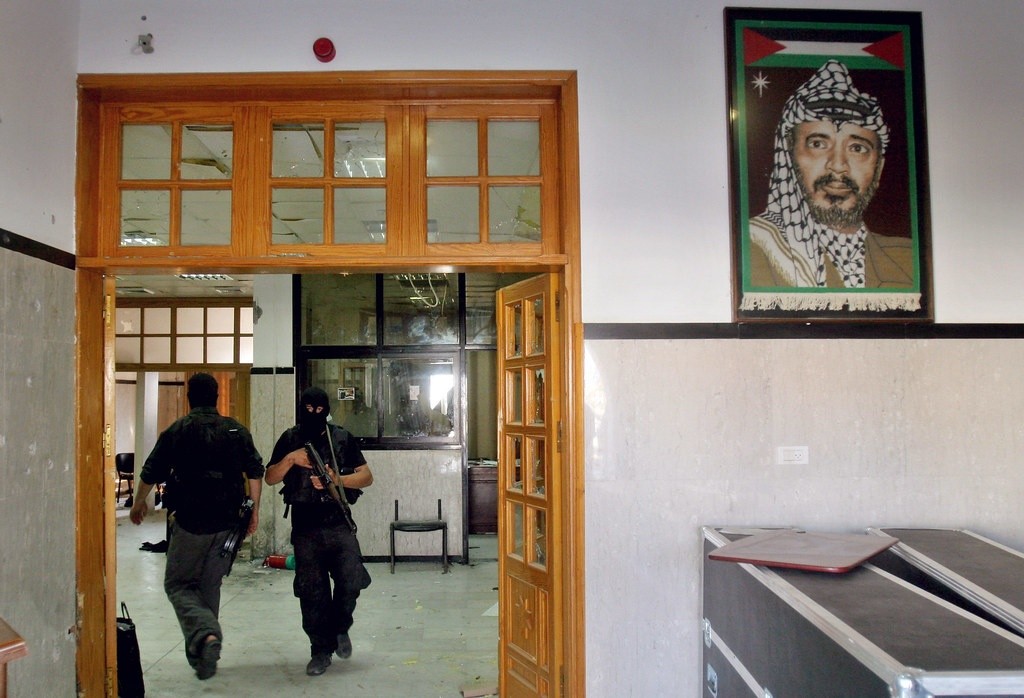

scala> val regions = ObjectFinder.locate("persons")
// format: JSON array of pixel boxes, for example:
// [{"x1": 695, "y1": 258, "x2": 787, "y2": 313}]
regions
[
  {"x1": 748, "y1": 58, "x2": 914, "y2": 288},
  {"x1": 130, "y1": 372, "x2": 265, "y2": 680},
  {"x1": 265, "y1": 387, "x2": 374, "y2": 676}
]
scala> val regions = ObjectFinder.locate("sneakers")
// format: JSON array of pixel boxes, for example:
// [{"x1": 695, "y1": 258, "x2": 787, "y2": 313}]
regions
[
  {"x1": 306, "y1": 652, "x2": 332, "y2": 675},
  {"x1": 335, "y1": 633, "x2": 352, "y2": 658}
]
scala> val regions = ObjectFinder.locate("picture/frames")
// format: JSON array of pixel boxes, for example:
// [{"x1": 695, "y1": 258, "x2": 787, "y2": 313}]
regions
[{"x1": 724, "y1": 5, "x2": 935, "y2": 326}]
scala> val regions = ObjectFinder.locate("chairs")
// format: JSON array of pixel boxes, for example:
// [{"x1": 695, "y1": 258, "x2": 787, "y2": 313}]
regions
[
  {"x1": 390, "y1": 499, "x2": 448, "y2": 574},
  {"x1": 115, "y1": 452, "x2": 160, "y2": 504}
]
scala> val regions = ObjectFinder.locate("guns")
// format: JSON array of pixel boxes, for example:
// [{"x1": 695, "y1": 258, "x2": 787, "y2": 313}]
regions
[
  {"x1": 294, "y1": 443, "x2": 357, "y2": 537},
  {"x1": 219, "y1": 497, "x2": 255, "y2": 561}
]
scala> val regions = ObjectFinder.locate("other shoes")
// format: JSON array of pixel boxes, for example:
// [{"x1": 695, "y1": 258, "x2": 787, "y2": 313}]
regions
[{"x1": 197, "y1": 635, "x2": 221, "y2": 679}]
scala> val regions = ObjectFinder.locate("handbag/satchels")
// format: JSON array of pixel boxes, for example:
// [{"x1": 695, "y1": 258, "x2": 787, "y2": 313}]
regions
[{"x1": 116, "y1": 601, "x2": 145, "y2": 698}]
